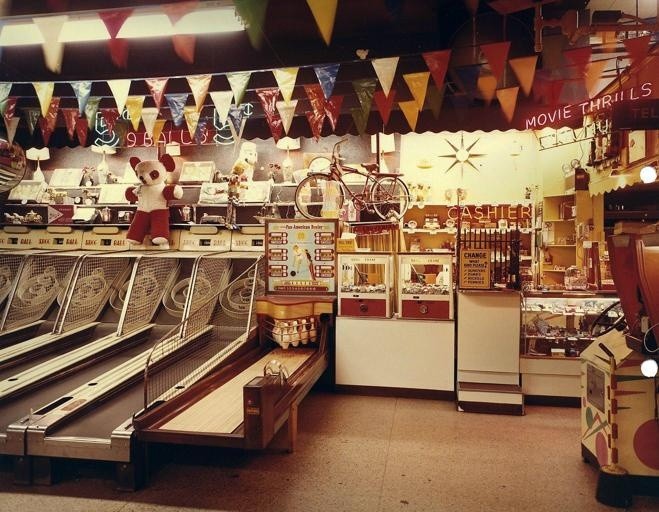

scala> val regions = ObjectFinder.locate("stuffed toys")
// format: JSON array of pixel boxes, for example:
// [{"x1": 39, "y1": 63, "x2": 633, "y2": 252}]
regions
[{"x1": 124, "y1": 154, "x2": 186, "y2": 248}]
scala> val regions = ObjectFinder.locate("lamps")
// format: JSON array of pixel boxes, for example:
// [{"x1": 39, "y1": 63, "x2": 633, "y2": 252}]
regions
[
  {"x1": 27, "y1": 147, "x2": 50, "y2": 182},
  {"x1": 91, "y1": 145, "x2": 116, "y2": 184},
  {"x1": 277, "y1": 136, "x2": 300, "y2": 183},
  {"x1": 371, "y1": 133, "x2": 395, "y2": 173},
  {"x1": 562, "y1": 159, "x2": 580, "y2": 178}
]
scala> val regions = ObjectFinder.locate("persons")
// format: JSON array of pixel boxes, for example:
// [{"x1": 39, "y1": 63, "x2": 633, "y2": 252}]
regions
[{"x1": 225, "y1": 162, "x2": 249, "y2": 231}]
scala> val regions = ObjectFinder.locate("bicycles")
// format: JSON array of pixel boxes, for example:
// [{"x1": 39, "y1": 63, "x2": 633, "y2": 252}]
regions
[{"x1": 293, "y1": 138, "x2": 412, "y2": 221}]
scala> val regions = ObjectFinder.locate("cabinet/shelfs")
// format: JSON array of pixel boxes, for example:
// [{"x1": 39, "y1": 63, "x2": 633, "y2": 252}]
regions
[
  {"x1": 540, "y1": 190, "x2": 604, "y2": 293},
  {"x1": 520, "y1": 290, "x2": 625, "y2": 399},
  {"x1": 1, "y1": 183, "x2": 405, "y2": 227},
  {"x1": 401, "y1": 199, "x2": 535, "y2": 288},
  {"x1": 398, "y1": 252, "x2": 454, "y2": 320},
  {"x1": 337, "y1": 252, "x2": 394, "y2": 318}
]
